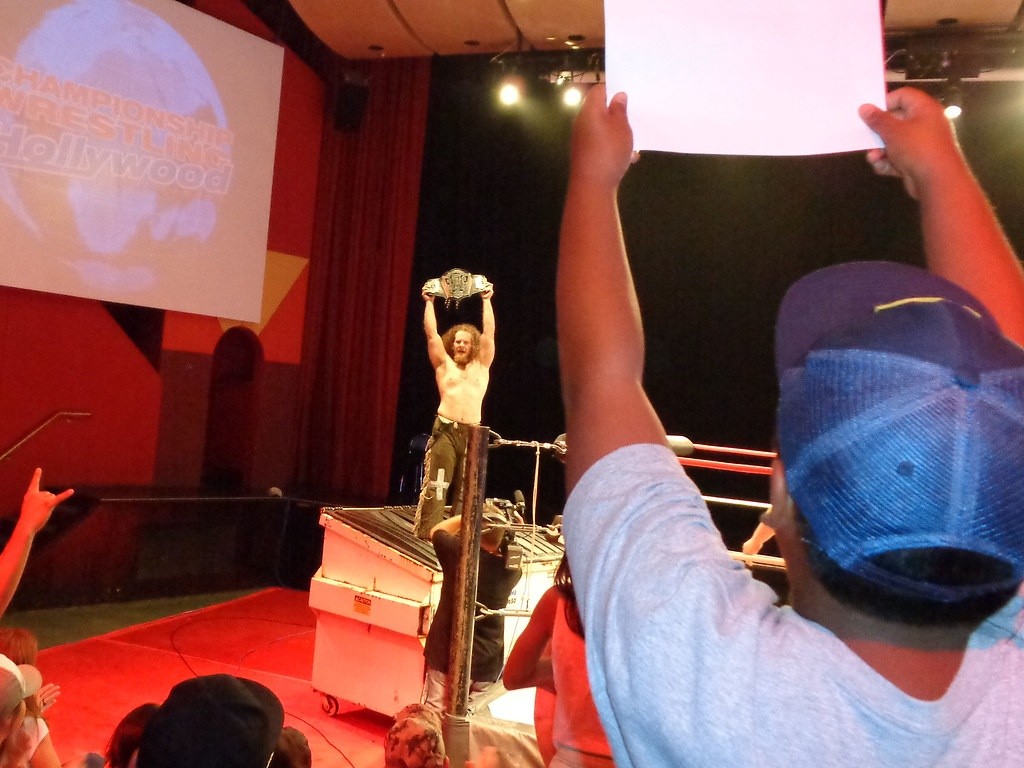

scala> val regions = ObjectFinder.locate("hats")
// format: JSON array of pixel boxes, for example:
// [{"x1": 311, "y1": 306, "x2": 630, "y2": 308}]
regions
[
  {"x1": 383, "y1": 703, "x2": 446, "y2": 768},
  {"x1": 136, "y1": 674, "x2": 284, "y2": 768},
  {"x1": 0, "y1": 654, "x2": 42, "y2": 718},
  {"x1": 775, "y1": 260, "x2": 1024, "y2": 622}
]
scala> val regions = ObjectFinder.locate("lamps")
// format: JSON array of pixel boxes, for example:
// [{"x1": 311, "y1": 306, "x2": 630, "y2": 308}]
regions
[{"x1": 943, "y1": 83, "x2": 964, "y2": 117}]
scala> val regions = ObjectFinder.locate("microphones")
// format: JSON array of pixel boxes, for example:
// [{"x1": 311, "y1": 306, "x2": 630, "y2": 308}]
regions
[{"x1": 514, "y1": 490, "x2": 526, "y2": 515}]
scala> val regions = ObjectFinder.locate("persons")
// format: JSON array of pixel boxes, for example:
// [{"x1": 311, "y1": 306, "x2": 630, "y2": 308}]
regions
[
  {"x1": 413, "y1": 282, "x2": 496, "y2": 539},
  {"x1": 742, "y1": 506, "x2": 776, "y2": 566},
  {"x1": 421, "y1": 503, "x2": 523, "y2": 709},
  {"x1": 556, "y1": 82, "x2": 1024, "y2": 768},
  {"x1": 384, "y1": 703, "x2": 450, "y2": 768},
  {"x1": 103, "y1": 673, "x2": 312, "y2": 768},
  {"x1": 502, "y1": 549, "x2": 615, "y2": 768},
  {"x1": 0, "y1": 468, "x2": 106, "y2": 768}
]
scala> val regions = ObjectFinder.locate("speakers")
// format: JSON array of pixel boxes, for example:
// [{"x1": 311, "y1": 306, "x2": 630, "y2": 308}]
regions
[{"x1": 339, "y1": 84, "x2": 366, "y2": 127}]
